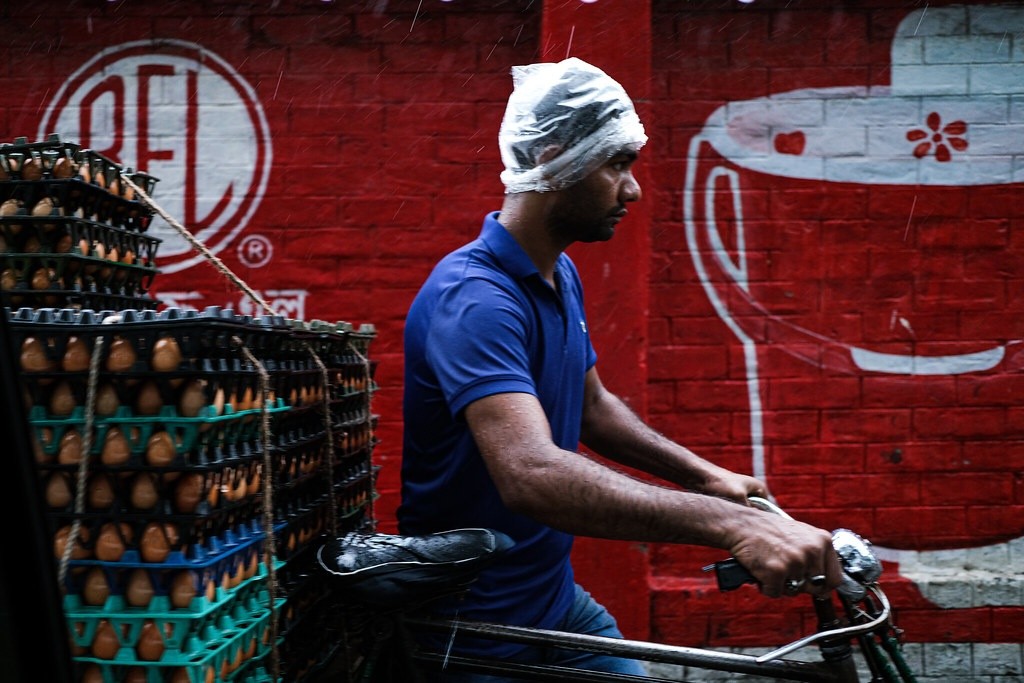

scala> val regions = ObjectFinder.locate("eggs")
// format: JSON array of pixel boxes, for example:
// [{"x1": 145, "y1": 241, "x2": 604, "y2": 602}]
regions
[
  {"x1": 18, "y1": 334, "x2": 369, "y2": 683},
  {"x1": 0, "y1": 153, "x2": 150, "y2": 304}
]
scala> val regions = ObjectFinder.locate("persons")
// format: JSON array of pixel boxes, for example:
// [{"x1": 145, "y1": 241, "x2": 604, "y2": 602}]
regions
[{"x1": 396, "y1": 57, "x2": 843, "y2": 683}]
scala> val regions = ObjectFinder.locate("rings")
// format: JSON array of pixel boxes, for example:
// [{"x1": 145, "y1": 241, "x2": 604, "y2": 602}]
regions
[
  {"x1": 809, "y1": 575, "x2": 826, "y2": 586},
  {"x1": 776, "y1": 574, "x2": 807, "y2": 596}
]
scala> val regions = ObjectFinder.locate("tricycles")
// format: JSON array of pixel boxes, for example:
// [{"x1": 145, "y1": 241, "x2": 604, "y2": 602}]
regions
[{"x1": 309, "y1": 491, "x2": 918, "y2": 683}]
